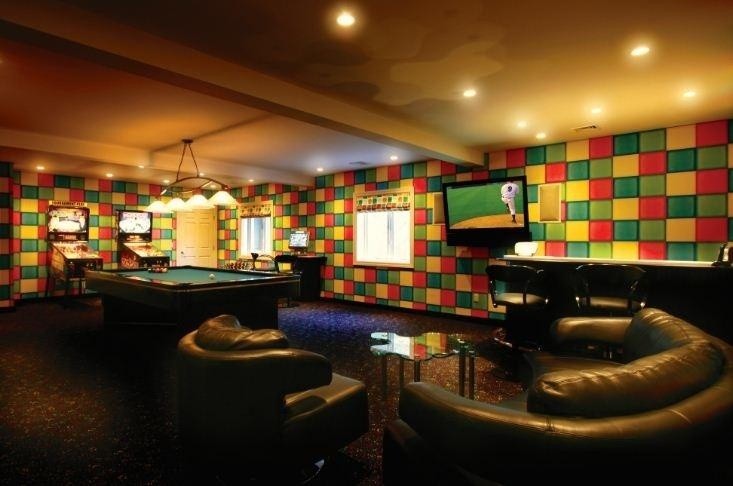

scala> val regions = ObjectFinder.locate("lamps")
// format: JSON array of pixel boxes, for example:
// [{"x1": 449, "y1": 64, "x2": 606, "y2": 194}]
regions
[{"x1": 143, "y1": 139, "x2": 241, "y2": 214}]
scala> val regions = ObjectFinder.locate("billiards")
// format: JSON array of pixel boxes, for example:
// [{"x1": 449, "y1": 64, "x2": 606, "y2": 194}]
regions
[
  {"x1": 210, "y1": 274, "x2": 215, "y2": 279},
  {"x1": 149, "y1": 267, "x2": 167, "y2": 273}
]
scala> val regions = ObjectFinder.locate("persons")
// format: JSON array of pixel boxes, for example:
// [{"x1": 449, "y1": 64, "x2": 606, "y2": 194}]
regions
[{"x1": 500, "y1": 180, "x2": 519, "y2": 223}]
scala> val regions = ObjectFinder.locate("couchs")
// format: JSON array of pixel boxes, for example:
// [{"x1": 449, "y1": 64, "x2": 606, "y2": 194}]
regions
[{"x1": 382, "y1": 308, "x2": 733, "y2": 486}]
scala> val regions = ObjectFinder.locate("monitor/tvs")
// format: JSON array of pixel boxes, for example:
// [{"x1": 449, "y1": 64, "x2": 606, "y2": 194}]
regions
[
  {"x1": 288, "y1": 234, "x2": 309, "y2": 248},
  {"x1": 441, "y1": 174, "x2": 529, "y2": 247}
]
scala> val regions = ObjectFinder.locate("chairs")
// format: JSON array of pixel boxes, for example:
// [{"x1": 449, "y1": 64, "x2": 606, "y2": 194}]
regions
[
  {"x1": 179, "y1": 314, "x2": 369, "y2": 486},
  {"x1": 485, "y1": 264, "x2": 550, "y2": 381},
  {"x1": 575, "y1": 264, "x2": 647, "y2": 360}
]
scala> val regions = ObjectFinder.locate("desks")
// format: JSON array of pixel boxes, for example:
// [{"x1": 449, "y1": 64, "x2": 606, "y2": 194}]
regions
[
  {"x1": 275, "y1": 255, "x2": 327, "y2": 300},
  {"x1": 86, "y1": 265, "x2": 300, "y2": 329}
]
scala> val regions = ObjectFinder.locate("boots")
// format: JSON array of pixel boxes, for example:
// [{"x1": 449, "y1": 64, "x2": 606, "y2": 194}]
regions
[{"x1": 511, "y1": 215, "x2": 516, "y2": 222}]
